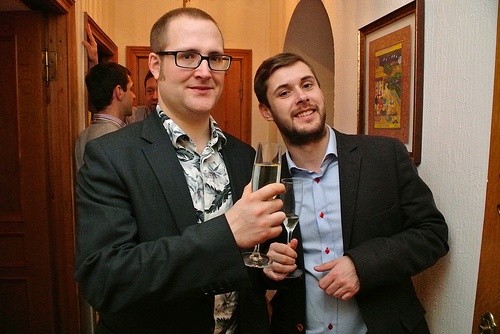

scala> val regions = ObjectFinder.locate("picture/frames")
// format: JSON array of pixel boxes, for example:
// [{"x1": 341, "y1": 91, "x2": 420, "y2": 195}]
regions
[{"x1": 355, "y1": 0, "x2": 426, "y2": 166}]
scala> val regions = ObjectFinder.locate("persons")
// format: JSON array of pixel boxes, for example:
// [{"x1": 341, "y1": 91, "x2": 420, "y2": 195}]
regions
[
  {"x1": 81, "y1": 23, "x2": 159, "y2": 126},
  {"x1": 249, "y1": 53, "x2": 447, "y2": 334},
  {"x1": 73, "y1": 7, "x2": 287, "y2": 334},
  {"x1": 75, "y1": 63, "x2": 136, "y2": 177}
]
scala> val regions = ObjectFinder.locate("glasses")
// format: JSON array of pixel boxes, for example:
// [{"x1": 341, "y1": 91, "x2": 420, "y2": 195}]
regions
[{"x1": 153, "y1": 51, "x2": 232, "y2": 71}]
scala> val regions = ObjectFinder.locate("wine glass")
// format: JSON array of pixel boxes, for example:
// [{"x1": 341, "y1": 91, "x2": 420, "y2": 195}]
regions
[
  {"x1": 281, "y1": 177, "x2": 303, "y2": 278},
  {"x1": 242, "y1": 142, "x2": 282, "y2": 268}
]
